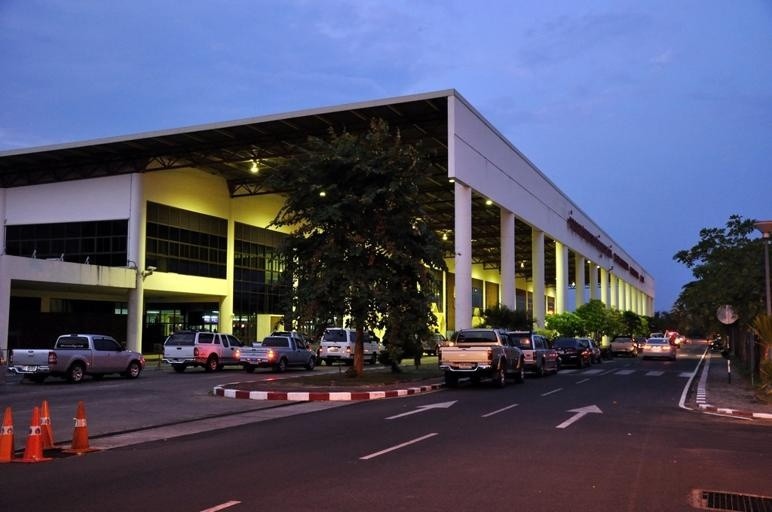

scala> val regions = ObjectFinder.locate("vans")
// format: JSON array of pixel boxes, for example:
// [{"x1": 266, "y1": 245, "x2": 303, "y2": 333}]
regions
[{"x1": 318, "y1": 328, "x2": 380, "y2": 367}]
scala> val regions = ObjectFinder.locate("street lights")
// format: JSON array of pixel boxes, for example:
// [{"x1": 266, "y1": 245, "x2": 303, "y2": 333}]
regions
[{"x1": 752, "y1": 220, "x2": 772, "y2": 316}]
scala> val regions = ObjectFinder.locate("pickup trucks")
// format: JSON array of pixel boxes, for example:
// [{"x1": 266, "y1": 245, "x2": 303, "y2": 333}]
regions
[
  {"x1": 438, "y1": 327, "x2": 527, "y2": 390},
  {"x1": 236, "y1": 334, "x2": 316, "y2": 373},
  {"x1": 7, "y1": 332, "x2": 147, "y2": 385}
]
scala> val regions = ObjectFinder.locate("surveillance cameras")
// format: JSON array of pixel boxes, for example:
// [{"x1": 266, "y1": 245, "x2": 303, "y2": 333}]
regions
[
  {"x1": 128, "y1": 261, "x2": 136, "y2": 269},
  {"x1": 147, "y1": 266, "x2": 157, "y2": 270}
]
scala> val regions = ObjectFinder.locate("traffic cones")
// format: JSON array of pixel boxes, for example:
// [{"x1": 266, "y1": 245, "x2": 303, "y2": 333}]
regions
[
  {"x1": 13, "y1": 406, "x2": 53, "y2": 463},
  {"x1": 39, "y1": 398, "x2": 61, "y2": 449},
  {"x1": 60, "y1": 399, "x2": 99, "y2": 454},
  {"x1": 0, "y1": 405, "x2": 18, "y2": 463}
]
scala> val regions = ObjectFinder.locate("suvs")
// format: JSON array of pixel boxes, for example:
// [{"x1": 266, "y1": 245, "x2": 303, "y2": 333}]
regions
[
  {"x1": 505, "y1": 329, "x2": 563, "y2": 378},
  {"x1": 160, "y1": 330, "x2": 254, "y2": 374},
  {"x1": 417, "y1": 333, "x2": 447, "y2": 356},
  {"x1": 270, "y1": 330, "x2": 311, "y2": 351}
]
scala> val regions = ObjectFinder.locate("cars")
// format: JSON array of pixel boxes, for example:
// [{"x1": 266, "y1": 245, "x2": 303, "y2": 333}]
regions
[
  {"x1": 641, "y1": 337, "x2": 678, "y2": 360},
  {"x1": 551, "y1": 337, "x2": 592, "y2": 370},
  {"x1": 609, "y1": 337, "x2": 638, "y2": 357},
  {"x1": 578, "y1": 338, "x2": 601, "y2": 364},
  {"x1": 636, "y1": 329, "x2": 693, "y2": 349}
]
